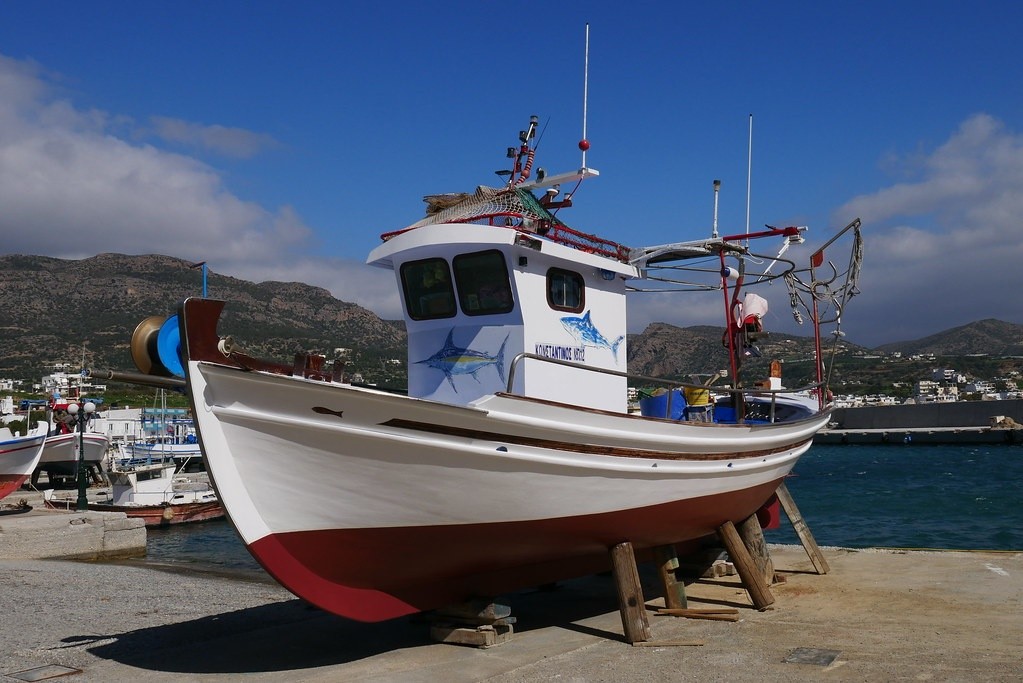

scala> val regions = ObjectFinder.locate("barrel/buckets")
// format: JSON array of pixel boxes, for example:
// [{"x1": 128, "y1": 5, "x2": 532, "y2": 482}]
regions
[
  {"x1": 686, "y1": 387, "x2": 709, "y2": 404},
  {"x1": 683, "y1": 405, "x2": 714, "y2": 424}
]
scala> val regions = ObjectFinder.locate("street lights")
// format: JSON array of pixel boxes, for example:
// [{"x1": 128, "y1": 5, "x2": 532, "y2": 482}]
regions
[{"x1": 66, "y1": 401, "x2": 95, "y2": 512}]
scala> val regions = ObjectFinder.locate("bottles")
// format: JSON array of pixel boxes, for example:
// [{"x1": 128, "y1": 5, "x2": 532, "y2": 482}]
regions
[{"x1": 722, "y1": 266, "x2": 739, "y2": 280}]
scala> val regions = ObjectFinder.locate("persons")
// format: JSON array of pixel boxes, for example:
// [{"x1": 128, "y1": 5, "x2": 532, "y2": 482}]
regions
[
  {"x1": 60, "y1": 415, "x2": 77, "y2": 434},
  {"x1": 53, "y1": 408, "x2": 67, "y2": 436}
]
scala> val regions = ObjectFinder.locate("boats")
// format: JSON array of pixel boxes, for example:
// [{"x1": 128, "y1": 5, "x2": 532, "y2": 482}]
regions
[
  {"x1": 80, "y1": 21, "x2": 864, "y2": 626},
  {"x1": 0, "y1": 420, "x2": 49, "y2": 501},
  {"x1": 26, "y1": 342, "x2": 225, "y2": 526}
]
